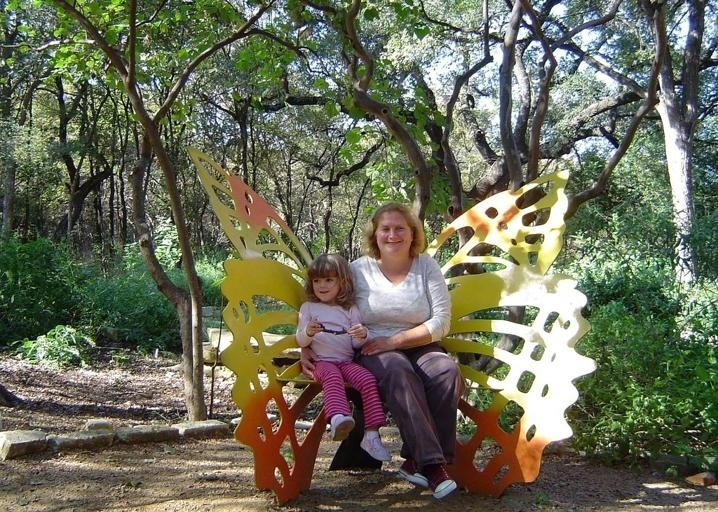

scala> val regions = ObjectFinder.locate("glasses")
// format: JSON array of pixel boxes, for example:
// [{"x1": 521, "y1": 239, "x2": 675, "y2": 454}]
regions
[{"x1": 318, "y1": 321, "x2": 348, "y2": 335}]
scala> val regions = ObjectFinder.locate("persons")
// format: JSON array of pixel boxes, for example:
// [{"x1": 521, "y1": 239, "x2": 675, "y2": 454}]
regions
[
  {"x1": 293, "y1": 253, "x2": 393, "y2": 463},
  {"x1": 299, "y1": 202, "x2": 465, "y2": 499}
]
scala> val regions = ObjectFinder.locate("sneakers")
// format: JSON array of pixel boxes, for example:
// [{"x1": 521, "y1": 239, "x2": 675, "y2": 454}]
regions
[
  {"x1": 359, "y1": 434, "x2": 391, "y2": 462},
  {"x1": 426, "y1": 465, "x2": 457, "y2": 501},
  {"x1": 398, "y1": 458, "x2": 429, "y2": 490},
  {"x1": 329, "y1": 416, "x2": 355, "y2": 441}
]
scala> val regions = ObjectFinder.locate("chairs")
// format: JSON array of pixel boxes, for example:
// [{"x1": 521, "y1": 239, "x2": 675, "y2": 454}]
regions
[{"x1": 183, "y1": 135, "x2": 596, "y2": 511}]
